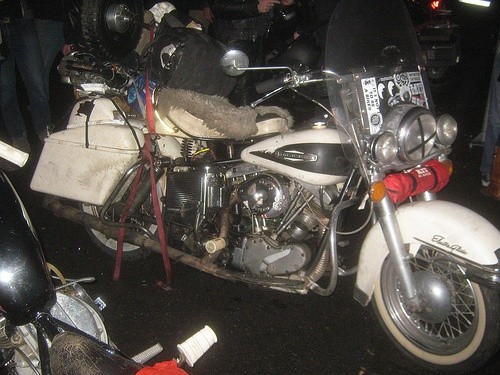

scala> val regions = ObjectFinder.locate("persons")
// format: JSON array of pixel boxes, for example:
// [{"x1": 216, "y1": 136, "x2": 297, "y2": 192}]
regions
[
  {"x1": 33, "y1": 0, "x2": 76, "y2": 111},
  {"x1": 0, "y1": 0, "x2": 56, "y2": 170},
  {"x1": 208, "y1": 0, "x2": 297, "y2": 104}
]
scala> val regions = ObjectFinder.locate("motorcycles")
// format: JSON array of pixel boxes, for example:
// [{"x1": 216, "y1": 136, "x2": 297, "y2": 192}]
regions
[{"x1": 30, "y1": 0, "x2": 500, "y2": 375}]
